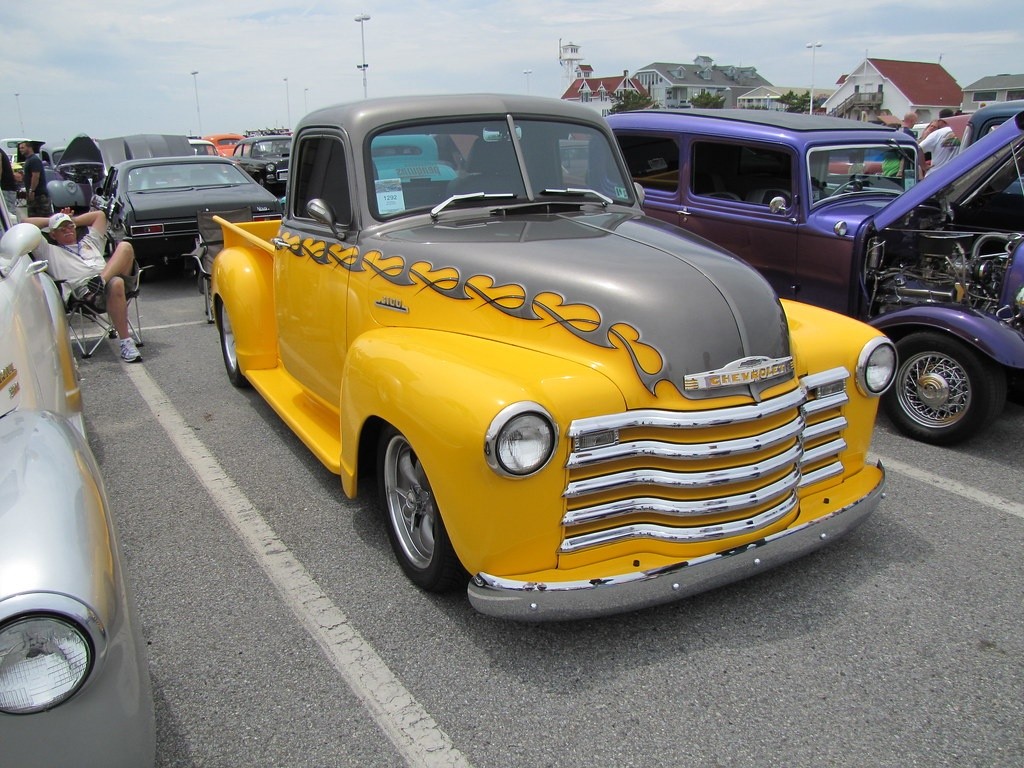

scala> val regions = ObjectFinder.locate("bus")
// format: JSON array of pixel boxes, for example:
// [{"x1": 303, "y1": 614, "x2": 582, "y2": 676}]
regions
[{"x1": 0, "y1": 138, "x2": 32, "y2": 163}]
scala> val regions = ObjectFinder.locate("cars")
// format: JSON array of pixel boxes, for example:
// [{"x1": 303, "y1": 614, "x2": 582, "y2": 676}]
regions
[
  {"x1": 280, "y1": 196, "x2": 286, "y2": 210},
  {"x1": 0, "y1": 188, "x2": 154, "y2": 768},
  {"x1": 605, "y1": 108, "x2": 1024, "y2": 446},
  {"x1": 39, "y1": 144, "x2": 68, "y2": 171},
  {"x1": 959, "y1": 99, "x2": 1024, "y2": 154},
  {"x1": 210, "y1": 92, "x2": 898, "y2": 621},
  {"x1": 201, "y1": 132, "x2": 251, "y2": 158},
  {"x1": 96, "y1": 134, "x2": 284, "y2": 283},
  {"x1": 187, "y1": 136, "x2": 218, "y2": 157}
]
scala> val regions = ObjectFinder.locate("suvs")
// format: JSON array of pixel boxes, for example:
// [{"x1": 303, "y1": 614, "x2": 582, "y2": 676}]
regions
[{"x1": 228, "y1": 129, "x2": 293, "y2": 193}]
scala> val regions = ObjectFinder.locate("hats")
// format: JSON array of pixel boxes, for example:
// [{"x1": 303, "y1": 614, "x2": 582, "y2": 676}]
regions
[{"x1": 48, "y1": 212, "x2": 72, "y2": 230}]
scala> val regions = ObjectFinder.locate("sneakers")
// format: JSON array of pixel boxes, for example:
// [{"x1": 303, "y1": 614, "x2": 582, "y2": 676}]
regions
[
  {"x1": 89, "y1": 276, "x2": 106, "y2": 297},
  {"x1": 120, "y1": 339, "x2": 143, "y2": 362}
]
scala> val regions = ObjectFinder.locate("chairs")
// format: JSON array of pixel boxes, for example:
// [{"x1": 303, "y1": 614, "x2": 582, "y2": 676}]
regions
[
  {"x1": 448, "y1": 128, "x2": 555, "y2": 201},
  {"x1": 26, "y1": 227, "x2": 154, "y2": 358},
  {"x1": 751, "y1": 188, "x2": 793, "y2": 210},
  {"x1": 180, "y1": 206, "x2": 253, "y2": 324}
]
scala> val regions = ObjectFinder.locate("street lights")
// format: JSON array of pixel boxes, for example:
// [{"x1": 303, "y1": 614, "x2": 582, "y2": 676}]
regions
[
  {"x1": 354, "y1": 13, "x2": 371, "y2": 98},
  {"x1": 283, "y1": 78, "x2": 291, "y2": 130},
  {"x1": 191, "y1": 71, "x2": 203, "y2": 134},
  {"x1": 806, "y1": 41, "x2": 822, "y2": 116},
  {"x1": 304, "y1": 89, "x2": 309, "y2": 115},
  {"x1": 523, "y1": 70, "x2": 532, "y2": 94},
  {"x1": 15, "y1": 92, "x2": 26, "y2": 139}
]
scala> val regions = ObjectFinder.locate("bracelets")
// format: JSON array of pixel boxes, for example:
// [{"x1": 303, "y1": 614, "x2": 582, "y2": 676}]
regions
[{"x1": 899, "y1": 170, "x2": 903, "y2": 172}]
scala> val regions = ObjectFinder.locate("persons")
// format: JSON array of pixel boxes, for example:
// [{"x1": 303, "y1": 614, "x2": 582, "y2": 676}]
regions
[
  {"x1": 899, "y1": 112, "x2": 927, "y2": 173},
  {"x1": 20, "y1": 141, "x2": 52, "y2": 217},
  {"x1": 918, "y1": 120, "x2": 938, "y2": 180},
  {"x1": 20, "y1": 207, "x2": 143, "y2": 363},
  {"x1": 882, "y1": 157, "x2": 904, "y2": 185},
  {"x1": 15, "y1": 171, "x2": 23, "y2": 189},
  {"x1": 919, "y1": 109, "x2": 960, "y2": 166},
  {"x1": 42, "y1": 161, "x2": 63, "y2": 183},
  {"x1": 0, "y1": 148, "x2": 17, "y2": 215}
]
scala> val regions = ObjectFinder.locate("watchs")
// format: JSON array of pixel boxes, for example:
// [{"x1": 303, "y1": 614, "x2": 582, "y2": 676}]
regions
[{"x1": 29, "y1": 189, "x2": 35, "y2": 192}]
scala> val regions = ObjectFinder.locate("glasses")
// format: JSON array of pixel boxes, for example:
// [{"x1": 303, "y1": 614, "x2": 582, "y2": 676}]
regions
[{"x1": 55, "y1": 222, "x2": 77, "y2": 233}]
